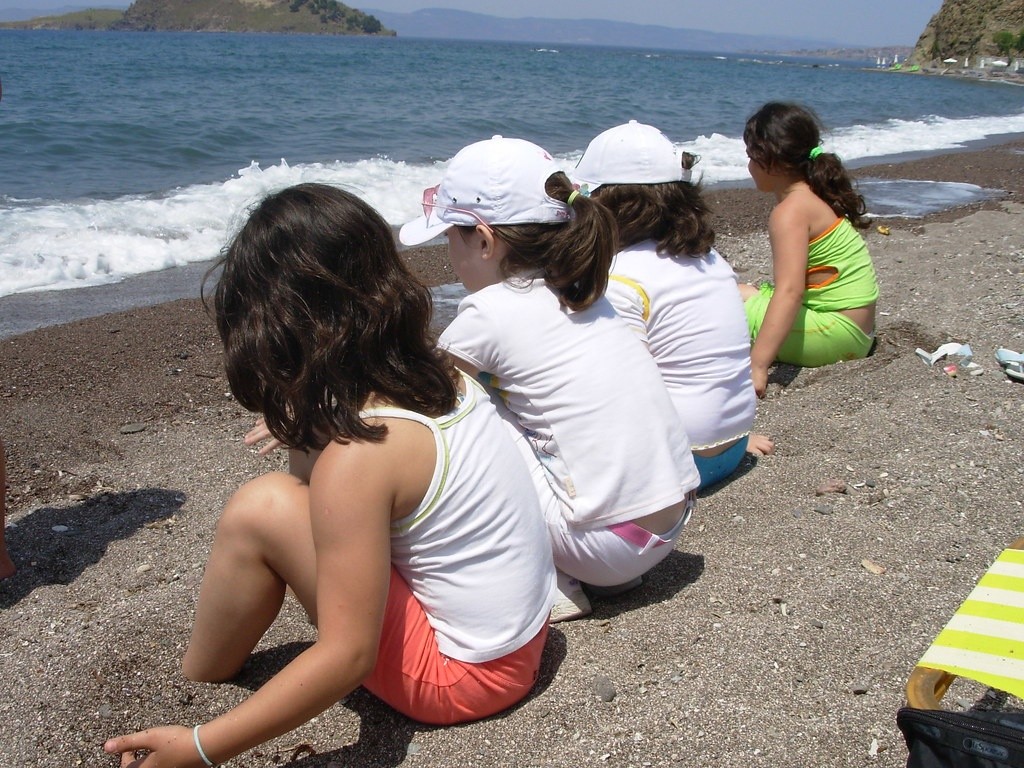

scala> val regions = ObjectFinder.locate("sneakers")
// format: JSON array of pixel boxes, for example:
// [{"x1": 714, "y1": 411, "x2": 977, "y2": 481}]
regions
[{"x1": 549, "y1": 569, "x2": 643, "y2": 625}]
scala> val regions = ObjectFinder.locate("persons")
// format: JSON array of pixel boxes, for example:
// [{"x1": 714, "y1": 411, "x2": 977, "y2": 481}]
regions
[
  {"x1": 104, "y1": 183, "x2": 558, "y2": 768},
  {"x1": 738, "y1": 101, "x2": 879, "y2": 398},
  {"x1": 567, "y1": 119, "x2": 775, "y2": 492},
  {"x1": 244, "y1": 135, "x2": 701, "y2": 624}
]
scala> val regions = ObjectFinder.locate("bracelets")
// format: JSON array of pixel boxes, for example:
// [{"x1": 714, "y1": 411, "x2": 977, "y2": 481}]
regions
[{"x1": 194, "y1": 725, "x2": 212, "y2": 766}]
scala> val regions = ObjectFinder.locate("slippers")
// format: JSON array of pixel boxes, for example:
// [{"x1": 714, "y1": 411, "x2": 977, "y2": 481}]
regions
[
  {"x1": 994, "y1": 349, "x2": 1024, "y2": 380},
  {"x1": 915, "y1": 342, "x2": 984, "y2": 378}
]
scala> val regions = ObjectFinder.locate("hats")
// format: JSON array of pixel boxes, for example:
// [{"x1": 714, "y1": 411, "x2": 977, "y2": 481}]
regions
[
  {"x1": 567, "y1": 120, "x2": 692, "y2": 197},
  {"x1": 399, "y1": 135, "x2": 576, "y2": 247}
]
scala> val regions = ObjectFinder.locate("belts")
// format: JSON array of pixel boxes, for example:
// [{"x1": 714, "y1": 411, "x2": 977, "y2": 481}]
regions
[{"x1": 605, "y1": 488, "x2": 694, "y2": 549}]
